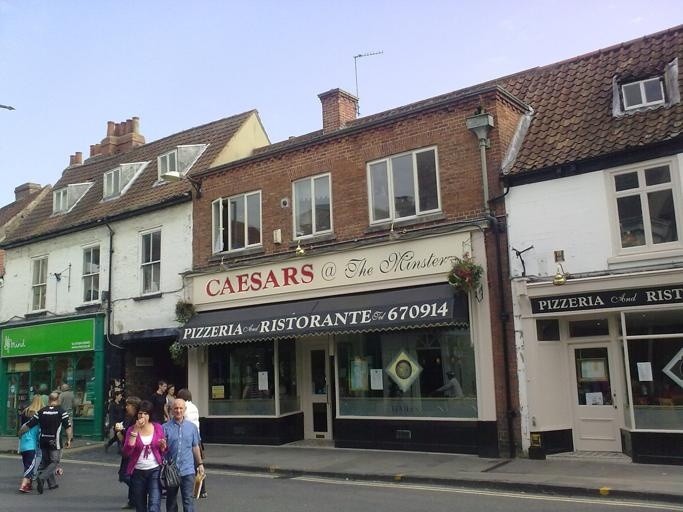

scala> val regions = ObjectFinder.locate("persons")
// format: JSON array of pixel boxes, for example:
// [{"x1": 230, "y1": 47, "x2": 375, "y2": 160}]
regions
[
  {"x1": 16, "y1": 390, "x2": 70, "y2": 494},
  {"x1": 435, "y1": 370, "x2": 464, "y2": 400},
  {"x1": 163, "y1": 384, "x2": 178, "y2": 419},
  {"x1": 112, "y1": 396, "x2": 141, "y2": 510},
  {"x1": 57, "y1": 384, "x2": 79, "y2": 438},
  {"x1": 159, "y1": 398, "x2": 206, "y2": 511},
  {"x1": 148, "y1": 381, "x2": 166, "y2": 426},
  {"x1": 102, "y1": 390, "x2": 126, "y2": 455},
  {"x1": 16, "y1": 394, "x2": 46, "y2": 493},
  {"x1": 121, "y1": 401, "x2": 166, "y2": 511},
  {"x1": 176, "y1": 389, "x2": 208, "y2": 499}
]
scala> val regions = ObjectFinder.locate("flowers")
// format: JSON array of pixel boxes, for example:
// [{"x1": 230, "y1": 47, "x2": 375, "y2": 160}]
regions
[{"x1": 448, "y1": 252, "x2": 484, "y2": 292}]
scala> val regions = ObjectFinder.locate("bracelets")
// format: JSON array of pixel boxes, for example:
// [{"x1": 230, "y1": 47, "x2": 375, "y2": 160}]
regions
[
  {"x1": 195, "y1": 461, "x2": 205, "y2": 467},
  {"x1": 128, "y1": 431, "x2": 138, "y2": 437}
]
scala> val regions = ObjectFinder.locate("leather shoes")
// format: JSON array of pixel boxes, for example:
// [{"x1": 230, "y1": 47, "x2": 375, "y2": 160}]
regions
[
  {"x1": 121, "y1": 504, "x2": 133, "y2": 509},
  {"x1": 36, "y1": 476, "x2": 44, "y2": 494},
  {"x1": 49, "y1": 485, "x2": 58, "y2": 490}
]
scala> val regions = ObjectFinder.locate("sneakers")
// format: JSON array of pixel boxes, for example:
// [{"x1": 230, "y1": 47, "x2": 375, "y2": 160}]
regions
[{"x1": 18, "y1": 486, "x2": 30, "y2": 493}]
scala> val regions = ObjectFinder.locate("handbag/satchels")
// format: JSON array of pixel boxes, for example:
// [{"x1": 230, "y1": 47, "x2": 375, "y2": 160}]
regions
[
  {"x1": 193, "y1": 472, "x2": 207, "y2": 500},
  {"x1": 159, "y1": 446, "x2": 181, "y2": 490}
]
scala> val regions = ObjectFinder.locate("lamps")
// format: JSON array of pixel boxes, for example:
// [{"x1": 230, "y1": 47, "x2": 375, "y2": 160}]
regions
[{"x1": 552, "y1": 262, "x2": 569, "y2": 286}]
